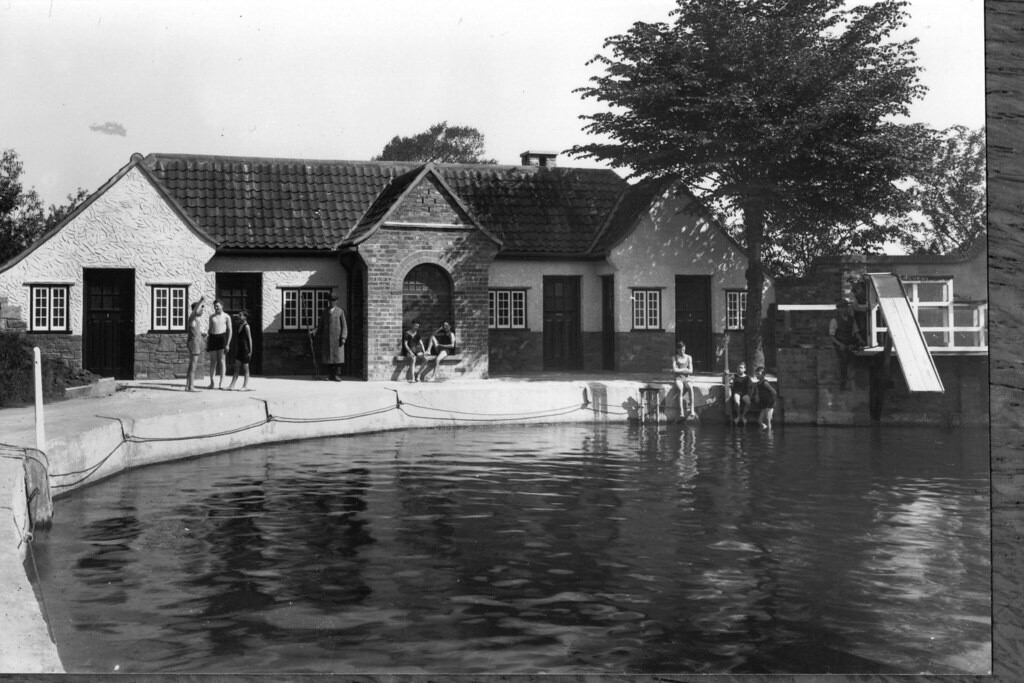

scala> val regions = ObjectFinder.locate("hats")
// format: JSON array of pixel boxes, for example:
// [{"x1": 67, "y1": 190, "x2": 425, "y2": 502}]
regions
[{"x1": 327, "y1": 292, "x2": 339, "y2": 301}]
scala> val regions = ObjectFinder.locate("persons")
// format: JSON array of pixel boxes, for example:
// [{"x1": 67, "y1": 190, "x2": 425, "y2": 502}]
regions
[
  {"x1": 425, "y1": 321, "x2": 455, "y2": 379},
  {"x1": 204, "y1": 299, "x2": 232, "y2": 389},
  {"x1": 225, "y1": 311, "x2": 252, "y2": 391},
  {"x1": 727, "y1": 362, "x2": 754, "y2": 424},
  {"x1": 672, "y1": 341, "x2": 696, "y2": 418},
  {"x1": 316, "y1": 292, "x2": 347, "y2": 381},
  {"x1": 185, "y1": 296, "x2": 206, "y2": 392},
  {"x1": 755, "y1": 366, "x2": 777, "y2": 432},
  {"x1": 829, "y1": 300, "x2": 863, "y2": 391},
  {"x1": 404, "y1": 320, "x2": 428, "y2": 382}
]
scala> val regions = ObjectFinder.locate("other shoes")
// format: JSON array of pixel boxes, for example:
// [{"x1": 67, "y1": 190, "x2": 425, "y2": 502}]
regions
[{"x1": 329, "y1": 374, "x2": 341, "y2": 381}]
